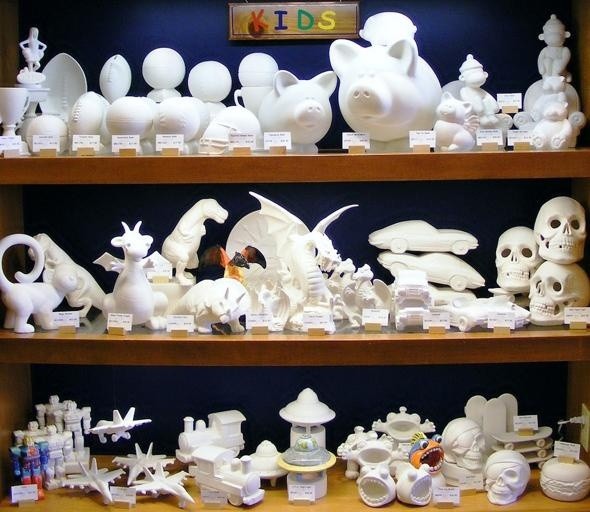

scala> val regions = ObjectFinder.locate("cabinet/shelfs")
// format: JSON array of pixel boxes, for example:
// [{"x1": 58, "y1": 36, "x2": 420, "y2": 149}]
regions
[{"x1": 0, "y1": 1, "x2": 590, "y2": 512}]
